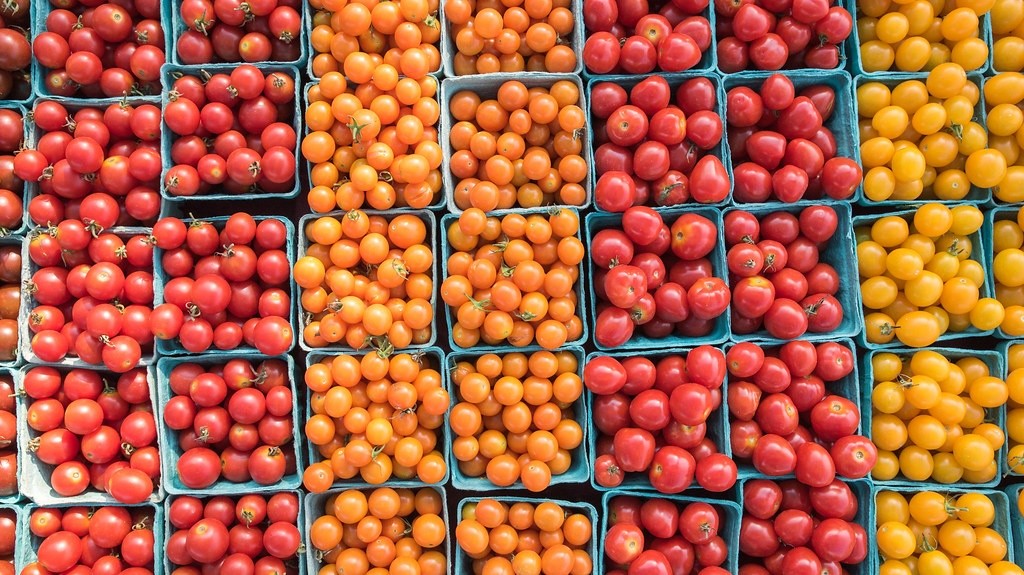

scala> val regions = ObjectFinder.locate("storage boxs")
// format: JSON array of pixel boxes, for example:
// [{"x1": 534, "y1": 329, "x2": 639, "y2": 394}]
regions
[{"x1": 0, "y1": 0, "x2": 1024, "y2": 575}]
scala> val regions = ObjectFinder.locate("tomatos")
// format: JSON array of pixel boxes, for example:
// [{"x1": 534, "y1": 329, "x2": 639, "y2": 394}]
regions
[{"x1": 0, "y1": 0, "x2": 1024, "y2": 575}]
